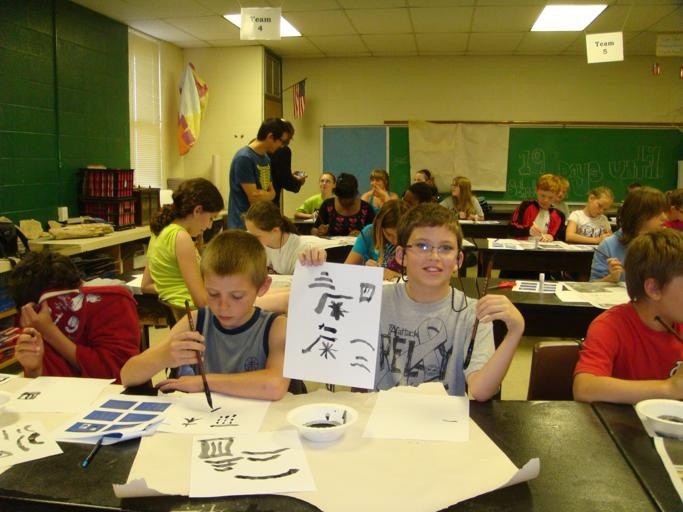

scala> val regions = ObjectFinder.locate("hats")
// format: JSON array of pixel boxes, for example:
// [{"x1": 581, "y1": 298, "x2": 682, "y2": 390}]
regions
[{"x1": 333, "y1": 174, "x2": 359, "y2": 196}]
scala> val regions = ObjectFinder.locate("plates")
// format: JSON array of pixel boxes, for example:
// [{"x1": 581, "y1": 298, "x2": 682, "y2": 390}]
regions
[{"x1": 634, "y1": 397, "x2": 683, "y2": 438}]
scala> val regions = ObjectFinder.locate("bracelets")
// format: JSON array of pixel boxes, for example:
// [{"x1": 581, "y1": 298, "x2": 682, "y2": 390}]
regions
[{"x1": 465, "y1": 213, "x2": 469, "y2": 220}]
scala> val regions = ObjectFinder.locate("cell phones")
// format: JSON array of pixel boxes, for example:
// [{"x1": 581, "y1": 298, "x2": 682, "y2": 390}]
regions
[{"x1": 297, "y1": 171, "x2": 304, "y2": 176}]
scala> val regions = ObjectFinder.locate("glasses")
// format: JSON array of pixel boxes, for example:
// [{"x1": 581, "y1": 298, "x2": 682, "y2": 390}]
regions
[{"x1": 405, "y1": 243, "x2": 456, "y2": 256}]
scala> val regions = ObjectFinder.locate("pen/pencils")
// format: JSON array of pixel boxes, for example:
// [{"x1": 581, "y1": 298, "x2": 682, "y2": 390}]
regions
[{"x1": 81, "y1": 434, "x2": 105, "y2": 467}]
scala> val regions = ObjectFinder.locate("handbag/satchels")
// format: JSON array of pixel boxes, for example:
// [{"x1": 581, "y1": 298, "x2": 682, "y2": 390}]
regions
[{"x1": 0, "y1": 221, "x2": 19, "y2": 258}]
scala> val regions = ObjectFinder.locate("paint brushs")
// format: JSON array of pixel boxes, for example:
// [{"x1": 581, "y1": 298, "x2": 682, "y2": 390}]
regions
[{"x1": 180, "y1": 296, "x2": 217, "y2": 411}]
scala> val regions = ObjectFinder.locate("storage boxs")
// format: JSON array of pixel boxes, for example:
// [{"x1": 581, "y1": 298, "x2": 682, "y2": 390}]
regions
[{"x1": 79, "y1": 168, "x2": 161, "y2": 231}]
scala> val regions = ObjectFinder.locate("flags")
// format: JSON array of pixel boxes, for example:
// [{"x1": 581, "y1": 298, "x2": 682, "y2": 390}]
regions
[{"x1": 292, "y1": 81, "x2": 305, "y2": 119}]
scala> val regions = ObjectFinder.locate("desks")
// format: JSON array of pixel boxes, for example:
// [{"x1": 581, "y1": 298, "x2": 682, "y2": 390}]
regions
[{"x1": 0, "y1": 210, "x2": 683, "y2": 512}]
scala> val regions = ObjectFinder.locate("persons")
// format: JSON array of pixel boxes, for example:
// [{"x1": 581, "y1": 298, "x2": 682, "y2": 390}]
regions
[
  {"x1": 564, "y1": 186, "x2": 614, "y2": 281},
  {"x1": 572, "y1": 228, "x2": 683, "y2": 403},
  {"x1": 300, "y1": 202, "x2": 525, "y2": 399},
  {"x1": 361, "y1": 168, "x2": 398, "y2": 214},
  {"x1": 296, "y1": 172, "x2": 336, "y2": 235},
  {"x1": 226, "y1": 117, "x2": 288, "y2": 230},
  {"x1": 440, "y1": 177, "x2": 484, "y2": 221},
  {"x1": 403, "y1": 183, "x2": 433, "y2": 207},
  {"x1": 509, "y1": 173, "x2": 566, "y2": 281},
  {"x1": 120, "y1": 229, "x2": 292, "y2": 401},
  {"x1": 345, "y1": 200, "x2": 412, "y2": 281},
  {"x1": 141, "y1": 178, "x2": 224, "y2": 322},
  {"x1": 402, "y1": 169, "x2": 440, "y2": 203},
  {"x1": 249, "y1": 119, "x2": 309, "y2": 208},
  {"x1": 591, "y1": 186, "x2": 672, "y2": 283},
  {"x1": 552, "y1": 177, "x2": 571, "y2": 217},
  {"x1": 661, "y1": 189, "x2": 683, "y2": 232},
  {"x1": 241, "y1": 200, "x2": 315, "y2": 275},
  {"x1": 312, "y1": 173, "x2": 376, "y2": 263},
  {"x1": 7, "y1": 250, "x2": 142, "y2": 380}
]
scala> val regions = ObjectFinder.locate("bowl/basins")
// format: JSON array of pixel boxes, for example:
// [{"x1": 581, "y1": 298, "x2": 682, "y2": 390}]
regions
[
  {"x1": 332, "y1": 421, "x2": 338, "y2": 422},
  {"x1": 285, "y1": 403, "x2": 358, "y2": 442}
]
